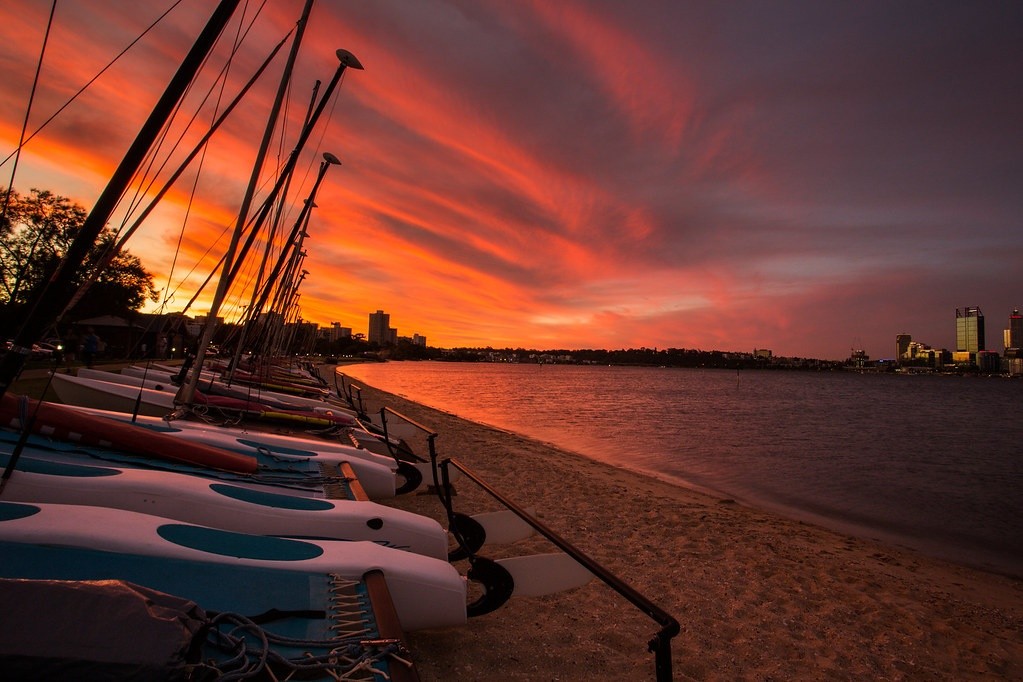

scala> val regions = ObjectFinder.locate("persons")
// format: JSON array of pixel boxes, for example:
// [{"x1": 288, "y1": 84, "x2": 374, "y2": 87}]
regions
[
  {"x1": 142, "y1": 342, "x2": 147, "y2": 360},
  {"x1": 61, "y1": 325, "x2": 108, "y2": 374},
  {"x1": 159, "y1": 335, "x2": 167, "y2": 361}
]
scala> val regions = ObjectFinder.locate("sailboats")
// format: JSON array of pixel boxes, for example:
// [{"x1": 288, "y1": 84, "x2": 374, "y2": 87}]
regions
[{"x1": 1, "y1": 1, "x2": 597, "y2": 681}]
